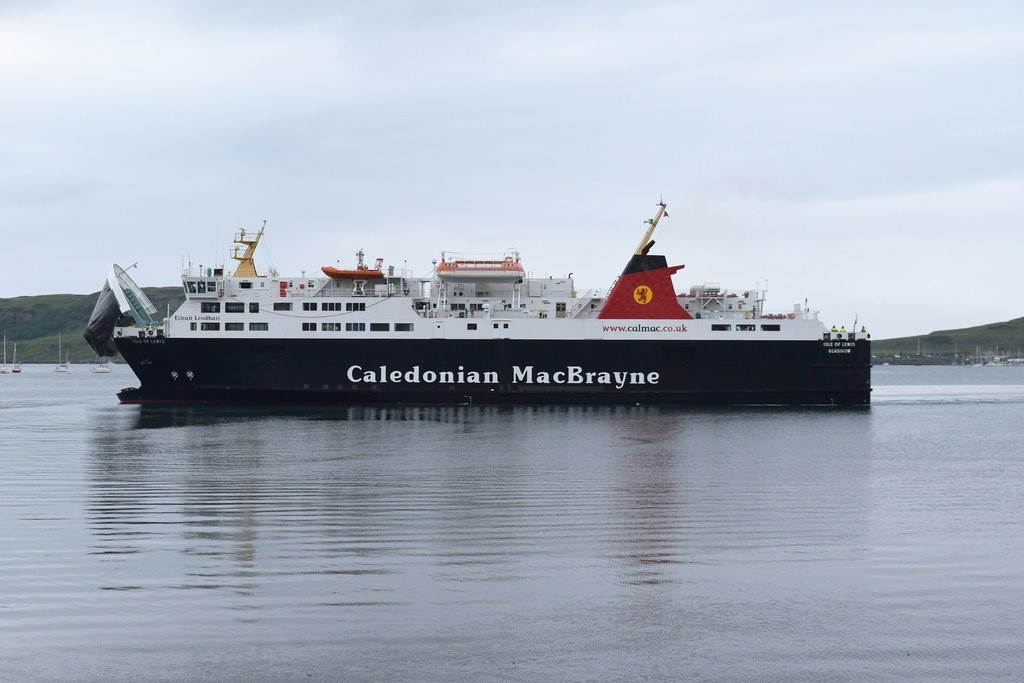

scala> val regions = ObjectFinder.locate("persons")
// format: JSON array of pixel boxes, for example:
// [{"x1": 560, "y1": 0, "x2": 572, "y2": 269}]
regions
[
  {"x1": 127, "y1": 321, "x2": 164, "y2": 329},
  {"x1": 831, "y1": 325, "x2": 838, "y2": 332},
  {"x1": 840, "y1": 326, "x2": 847, "y2": 332}
]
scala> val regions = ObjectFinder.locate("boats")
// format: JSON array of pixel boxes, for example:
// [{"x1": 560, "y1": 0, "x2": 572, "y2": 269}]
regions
[
  {"x1": 84, "y1": 201, "x2": 871, "y2": 405},
  {"x1": 438, "y1": 260, "x2": 525, "y2": 282},
  {"x1": 320, "y1": 266, "x2": 382, "y2": 279}
]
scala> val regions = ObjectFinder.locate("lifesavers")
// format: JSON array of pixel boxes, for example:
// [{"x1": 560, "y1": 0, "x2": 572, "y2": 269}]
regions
[{"x1": 789, "y1": 313, "x2": 795, "y2": 319}]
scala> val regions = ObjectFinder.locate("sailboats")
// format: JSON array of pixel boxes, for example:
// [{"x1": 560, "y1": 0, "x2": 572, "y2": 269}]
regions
[
  {"x1": 90, "y1": 354, "x2": 116, "y2": 373},
  {"x1": 12, "y1": 342, "x2": 22, "y2": 373},
  {"x1": 53, "y1": 331, "x2": 70, "y2": 372},
  {"x1": 0, "y1": 331, "x2": 10, "y2": 373},
  {"x1": 967, "y1": 346, "x2": 1024, "y2": 366}
]
